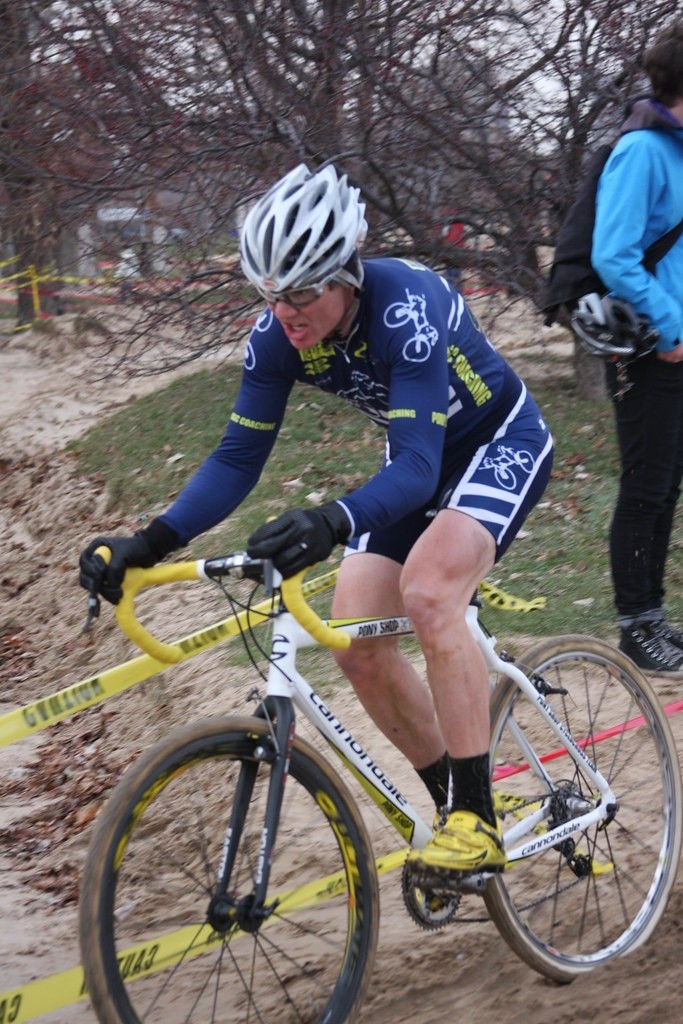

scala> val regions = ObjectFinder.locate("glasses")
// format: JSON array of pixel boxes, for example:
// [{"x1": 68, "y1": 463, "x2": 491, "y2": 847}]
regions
[{"x1": 256, "y1": 289, "x2": 321, "y2": 309}]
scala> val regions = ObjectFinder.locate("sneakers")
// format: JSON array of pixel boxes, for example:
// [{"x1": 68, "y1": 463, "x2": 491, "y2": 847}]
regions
[
  {"x1": 643, "y1": 617, "x2": 683, "y2": 649},
  {"x1": 422, "y1": 810, "x2": 456, "y2": 912},
  {"x1": 408, "y1": 810, "x2": 507, "y2": 877},
  {"x1": 618, "y1": 624, "x2": 683, "y2": 679}
]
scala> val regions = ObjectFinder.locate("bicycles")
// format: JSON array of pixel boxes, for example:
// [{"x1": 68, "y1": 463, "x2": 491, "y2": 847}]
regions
[{"x1": 78, "y1": 512, "x2": 681, "y2": 1024}]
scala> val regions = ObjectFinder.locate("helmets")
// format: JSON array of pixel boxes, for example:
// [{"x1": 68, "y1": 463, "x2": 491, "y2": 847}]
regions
[
  {"x1": 570, "y1": 290, "x2": 662, "y2": 362},
  {"x1": 239, "y1": 162, "x2": 369, "y2": 295}
]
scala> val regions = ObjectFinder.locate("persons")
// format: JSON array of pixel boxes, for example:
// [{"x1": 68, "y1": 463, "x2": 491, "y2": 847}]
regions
[
  {"x1": 79, "y1": 163, "x2": 554, "y2": 912},
  {"x1": 114, "y1": 242, "x2": 152, "y2": 303},
  {"x1": 589, "y1": 18, "x2": 683, "y2": 679},
  {"x1": 439, "y1": 206, "x2": 471, "y2": 293}
]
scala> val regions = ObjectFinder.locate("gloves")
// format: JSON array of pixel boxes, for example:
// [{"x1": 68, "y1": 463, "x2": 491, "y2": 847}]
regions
[
  {"x1": 77, "y1": 532, "x2": 154, "y2": 606},
  {"x1": 243, "y1": 499, "x2": 354, "y2": 584}
]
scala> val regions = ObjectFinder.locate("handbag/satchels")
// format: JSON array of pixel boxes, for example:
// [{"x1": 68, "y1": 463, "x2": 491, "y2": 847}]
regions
[{"x1": 542, "y1": 134, "x2": 683, "y2": 326}]
